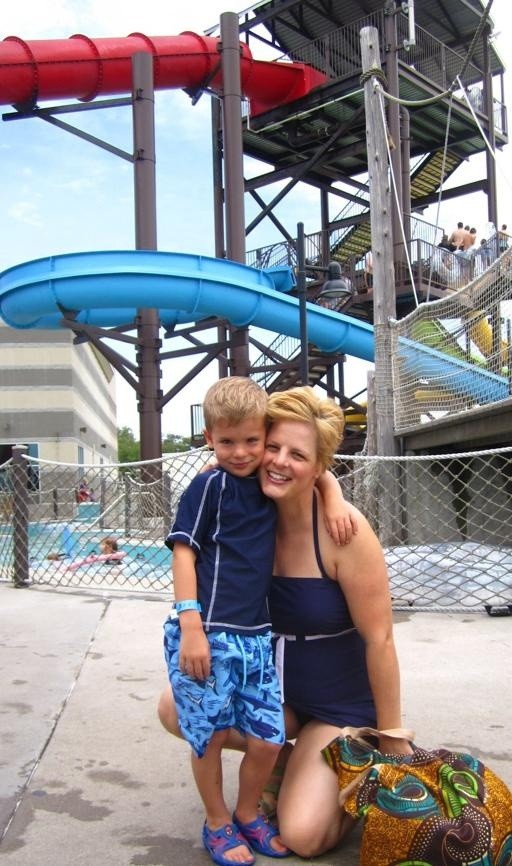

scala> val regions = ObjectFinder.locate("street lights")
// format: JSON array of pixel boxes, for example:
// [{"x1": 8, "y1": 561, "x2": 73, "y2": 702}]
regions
[{"x1": 296, "y1": 221, "x2": 351, "y2": 386}]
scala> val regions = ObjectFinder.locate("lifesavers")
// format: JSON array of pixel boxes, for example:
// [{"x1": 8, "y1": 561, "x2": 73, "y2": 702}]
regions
[
  {"x1": 434, "y1": 247, "x2": 460, "y2": 282},
  {"x1": 463, "y1": 86, "x2": 480, "y2": 103}
]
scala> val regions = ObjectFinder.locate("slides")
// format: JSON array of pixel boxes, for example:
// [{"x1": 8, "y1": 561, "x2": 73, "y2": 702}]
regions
[
  {"x1": 1, "y1": 250, "x2": 510, "y2": 425},
  {"x1": 2, "y1": 30, "x2": 324, "y2": 117}
]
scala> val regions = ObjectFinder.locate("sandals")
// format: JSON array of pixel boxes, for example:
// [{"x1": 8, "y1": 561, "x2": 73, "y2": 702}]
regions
[
  {"x1": 258, "y1": 765, "x2": 284, "y2": 819},
  {"x1": 232, "y1": 810, "x2": 294, "y2": 857},
  {"x1": 202, "y1": 818, "x2": 256, "y2": 866}
]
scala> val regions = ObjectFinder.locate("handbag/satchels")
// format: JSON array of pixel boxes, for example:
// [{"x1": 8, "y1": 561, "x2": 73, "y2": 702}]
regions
[{"x1": 320, "y1": 726, "x2": 512, "y2": 866}]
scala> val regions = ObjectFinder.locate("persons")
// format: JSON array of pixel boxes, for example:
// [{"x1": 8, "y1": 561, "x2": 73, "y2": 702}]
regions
[
  {"x1": 437, "y1": 222, "x2": 509, "y2": 271},
  {"x1": 78, "y1": 477, "x2": 96, "y2": 502},
  {"x1": 163, "y1": 375, "x2": 359, "y2": 866},
  {"x1": 101, "y1": 538, "x2": 122, "y2": 565},
  {"x1": 363, "y1": 249, "x2": 373, "y2": 294},
  {"x1": 157, "y1": 384, "x2": 413, "y2": 858}
]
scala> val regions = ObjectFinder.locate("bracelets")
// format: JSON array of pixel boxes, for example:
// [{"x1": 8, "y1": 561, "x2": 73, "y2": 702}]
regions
[{"x1": 176, "y1": 600, "x2": 203, "y2": 615}]
